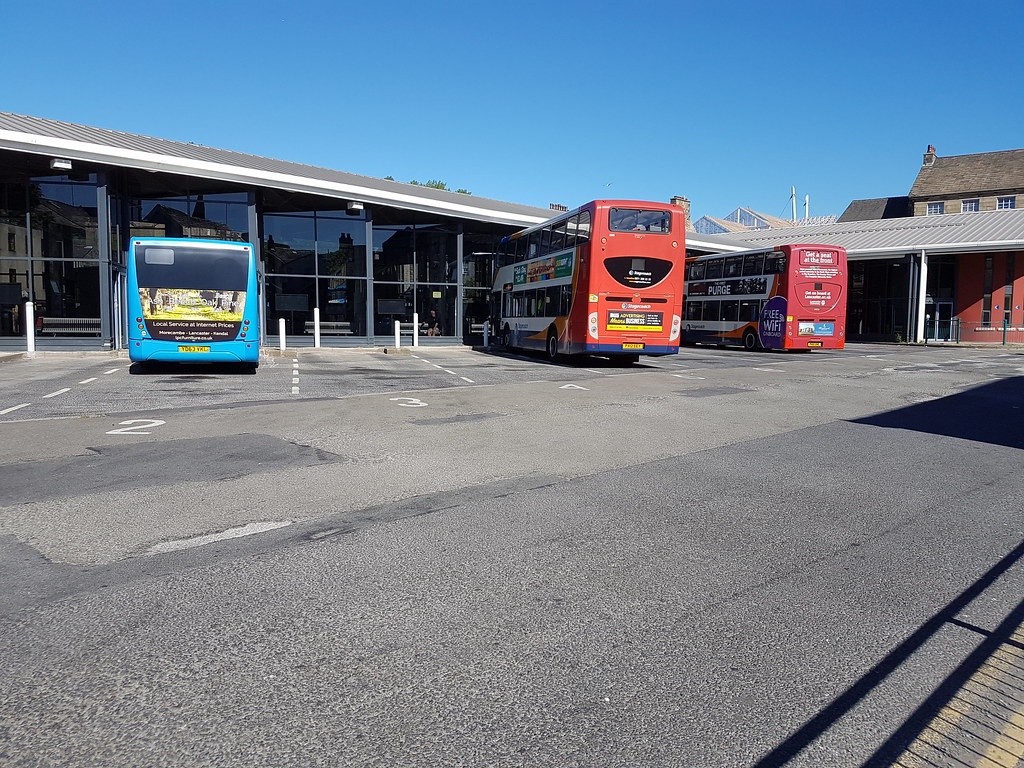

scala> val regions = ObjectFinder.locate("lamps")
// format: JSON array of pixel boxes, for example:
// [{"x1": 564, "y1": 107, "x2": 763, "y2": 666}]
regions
[
  {"x1": 348, "y1": 202, "x2": 364, "y2": 210},
  {"x1": 50, "y1": 159, "x2": 72, "y2": 170}
]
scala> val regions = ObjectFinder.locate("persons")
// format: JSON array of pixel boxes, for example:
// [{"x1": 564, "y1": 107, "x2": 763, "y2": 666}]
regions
[
  {"x1": 528, "y1": 265, "x2": 554, "y2": 282},
  {"x1": 422, "y1": 311, "x2": 439, "y2": 336}
]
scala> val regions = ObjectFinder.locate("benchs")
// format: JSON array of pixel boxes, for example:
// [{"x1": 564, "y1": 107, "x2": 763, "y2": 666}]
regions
[
  {"x1": 400, "y1": 323, "x2": 440, "y2": 335},
  {"x1": 42, "y1": 318, "x2": 101, "y2": 337},
  {"x1": 303, "y1": 321, "x2": 353, "y2": 335},
  {"x1": 471, "y1": 324, "x2": 491, "y2": 333}
]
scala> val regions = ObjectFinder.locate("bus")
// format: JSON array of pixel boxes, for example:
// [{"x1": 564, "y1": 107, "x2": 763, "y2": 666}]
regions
[
  {"x1": 127, "y1": 236, "x2": 262, "y2": 372},
  {"x1": 680, "y1": 245, "x2": 847, "y2": 352},
  {"x1": 491, "y1": 200, "x2": 685, "y2": 367}
]
